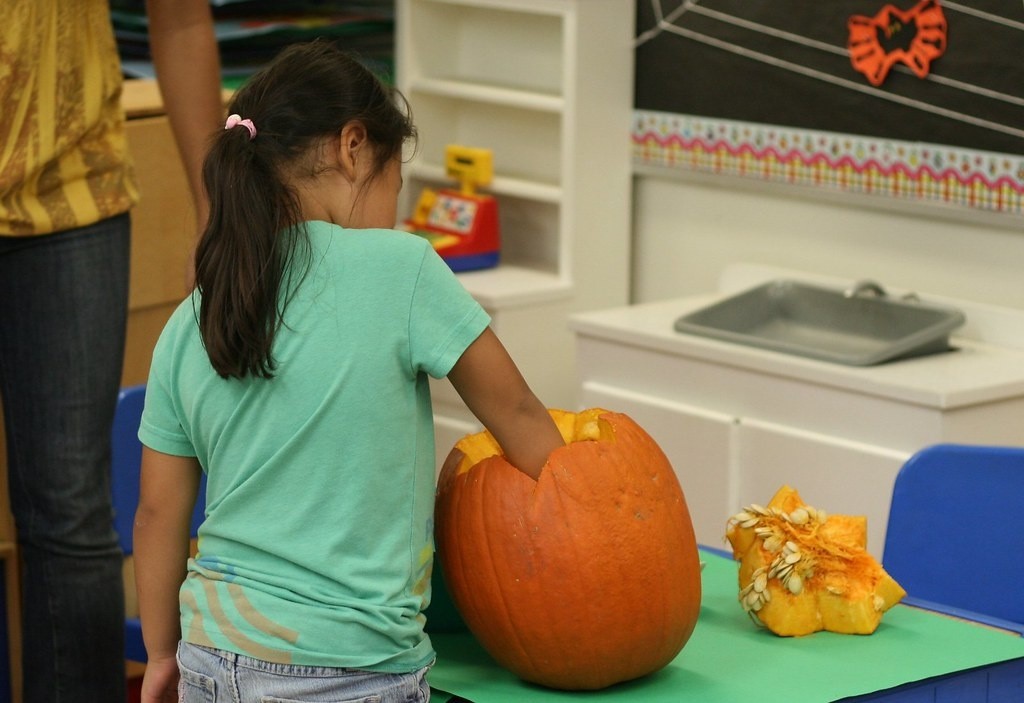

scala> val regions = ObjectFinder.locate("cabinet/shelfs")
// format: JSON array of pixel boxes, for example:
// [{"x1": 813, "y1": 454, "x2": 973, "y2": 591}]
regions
[
  {"x1": 391, "y1": 0, "x2": 637, "y2": 486},
  {"x1": 569, "y1": 293, "x2": 1023, "y2": 564}
]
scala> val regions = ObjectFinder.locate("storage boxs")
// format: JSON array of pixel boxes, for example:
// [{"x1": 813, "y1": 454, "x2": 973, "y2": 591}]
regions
[{"x1": 112, "y1": 78, "x2": 240, "y2": 683}]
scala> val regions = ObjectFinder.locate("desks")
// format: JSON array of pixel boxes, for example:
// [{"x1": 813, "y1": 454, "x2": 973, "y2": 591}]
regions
[{"x1": 418, "y1": 542, "x2": 1024, "y2": 702}]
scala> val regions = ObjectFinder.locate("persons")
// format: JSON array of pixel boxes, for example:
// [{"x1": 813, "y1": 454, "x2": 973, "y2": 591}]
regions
[
  {"x1": 133, "y1": 44, "x2": 566, "y2": 703},
  {"x1": 0, "y1": 0, "x2": 228, "y2": 703}
]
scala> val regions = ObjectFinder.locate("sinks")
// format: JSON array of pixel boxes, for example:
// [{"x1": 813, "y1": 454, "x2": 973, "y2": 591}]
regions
[{"x1": 672, "y1": 276, "x2": 964, "y2": 366}]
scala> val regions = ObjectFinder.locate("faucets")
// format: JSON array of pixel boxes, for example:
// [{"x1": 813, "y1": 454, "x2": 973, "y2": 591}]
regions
[{"x1": 844, "y1": 281, "x2": 887, "y2": 303}]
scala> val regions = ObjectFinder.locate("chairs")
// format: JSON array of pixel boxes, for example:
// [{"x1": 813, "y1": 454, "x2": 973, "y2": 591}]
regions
[
  {"x1": 109, "y1": 386, "x2": 208, "y2": 664},
  {"x1": 883, "y1": 442, "x2": 1024, "y2": 628}
]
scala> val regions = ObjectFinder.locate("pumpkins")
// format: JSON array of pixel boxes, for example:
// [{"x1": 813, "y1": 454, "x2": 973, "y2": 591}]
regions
[
  {"x1": 435, "y1": 407, "x2": 702, "y2": 694},
  {"x1": 725, "y1": 484, "x2": 908, "y2": 638}
]
